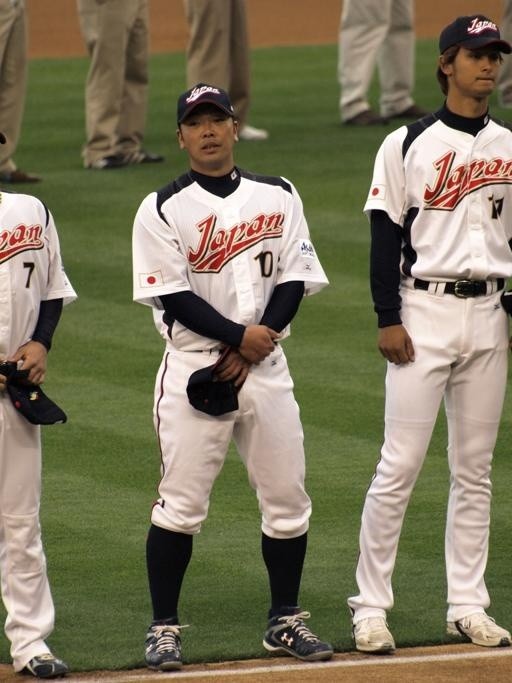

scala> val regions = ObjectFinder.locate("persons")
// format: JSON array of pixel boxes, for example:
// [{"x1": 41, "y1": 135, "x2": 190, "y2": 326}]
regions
[
  {"x1": 1, "y1": 1, "x2": 42, "y2": 183},
  {"x1": 181, "y1": 0, "x2": 269, "y2": 146},
  {"x1": 1, "y1": 130, "x2": 78, "y2": 677},
  {"x1": 131, "y1": 85, "x2": 335, "y2": 670},
  {"x1": 497, "y1": 1, "x2": 512, "y2": 109},
  {"x1": 76, "y1": 0, "x2": 165, "y2": 168},
  {"x1": 346, "y1": 12, "x2": 512, "y2": 654},
  {"x1": 336, "y1": 0, "x2": 426, "y2": 127}
]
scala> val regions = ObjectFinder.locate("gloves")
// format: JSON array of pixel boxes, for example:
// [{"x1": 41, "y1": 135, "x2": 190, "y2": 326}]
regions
[{"x1": 6, "y1": 370, "x2": 69, "y2": 427}]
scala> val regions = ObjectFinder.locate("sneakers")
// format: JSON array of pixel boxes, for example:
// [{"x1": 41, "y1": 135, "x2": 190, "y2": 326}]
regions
[
  {"x1": 344, "y1": 106, "x2": 391, "y2": 128},
  {"x1": 238, "y1": 121, "x2": 271, "y2": 142},
  {"x1": 444, "y1": 607, "x2": 512, "y2": 648},
  {"x1": 84, "y1": 150, "x2": 129, "y2": 171},
  {"x1": 261, "y1": 604, "x2": 335, "y2": 662},
  {"x1": 0, "y1": 165, "x2": 43, "y2": 185},
  {"x1": 123, "y1": 147, "x2": 165, "y2": 166},
  {"x1": 14, "y1": 650, "x2": 72, "y2": 680},
  {"x1": 141, "y1": 617, "x2": 187, "y2": 672},
  {"x1": 350, "y1": 609, "x2": 397, "y2": 654},
  {"x1": 381, "y1": 101, "x2": 431, "y2": 122}
]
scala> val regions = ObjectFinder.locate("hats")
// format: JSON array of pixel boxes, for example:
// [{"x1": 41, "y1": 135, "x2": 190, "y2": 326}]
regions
[
  {"x1": 437, "y1": 11, "x2": 511, "y2": 61},
  {"x1": 175, "y1": 79, "x2": 235, "y2": 125},
  {"x1": 184, "y1": 343, "x2": 242, "y2": 417}
]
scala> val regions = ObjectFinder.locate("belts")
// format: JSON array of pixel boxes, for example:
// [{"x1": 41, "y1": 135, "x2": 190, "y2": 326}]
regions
[
  {"x1": 399, "y1": 273, "x2": 507, "y2": 301},
  {"x1": 0, "y1": 359, "x2": 32, "y2": 378}
]
